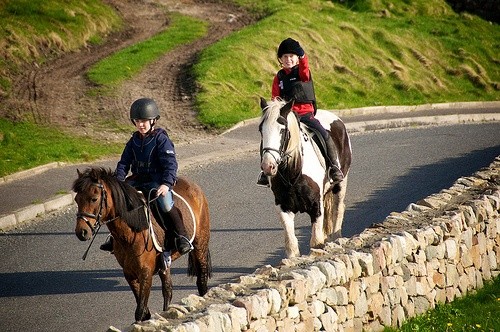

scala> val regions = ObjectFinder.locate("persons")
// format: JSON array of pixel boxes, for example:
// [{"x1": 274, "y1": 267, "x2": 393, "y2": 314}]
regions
[
  {"x1": 257, "y1": 38, "x2": 343, "y2": 185},
  {"x1": 100, "y1": 98, "x2": 190, "y2": 254}
]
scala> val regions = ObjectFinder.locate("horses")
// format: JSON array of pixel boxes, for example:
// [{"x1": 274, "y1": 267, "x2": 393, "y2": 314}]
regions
[
  {"x1": 258, "y1": 94, "x2": 352, "y2": 259},
  {"x1": 72, "y1": 167, "x2": 213, "y2": 324}
]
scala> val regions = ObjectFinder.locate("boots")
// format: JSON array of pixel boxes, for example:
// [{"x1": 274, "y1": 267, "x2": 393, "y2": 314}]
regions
[
  {"x1": 326, "y1": 134, "x2": 343, "y2": 180},
  {"x1": 256, "y1": 172, "x2": 268, "y2": 185},
  {"x1": 100, "y1": 235, "x2": 114, "y2": 251},
  {"x1": 166, "y1": 206, "x2": 191, "y2": 254}
]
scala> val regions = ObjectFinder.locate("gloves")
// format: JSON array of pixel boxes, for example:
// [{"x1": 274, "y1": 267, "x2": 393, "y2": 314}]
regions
[{"x1": 294, "y1": 42, "x2": 304, "y2": 56}]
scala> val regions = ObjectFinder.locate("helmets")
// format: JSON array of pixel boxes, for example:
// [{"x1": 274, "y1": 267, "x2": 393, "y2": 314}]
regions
[
  {"x1": 129, "y1": 97, "x2": 161, "y2": 120},
  {"x1": 277, "y1": 37, "x2": 300, "y2": 58}
]
